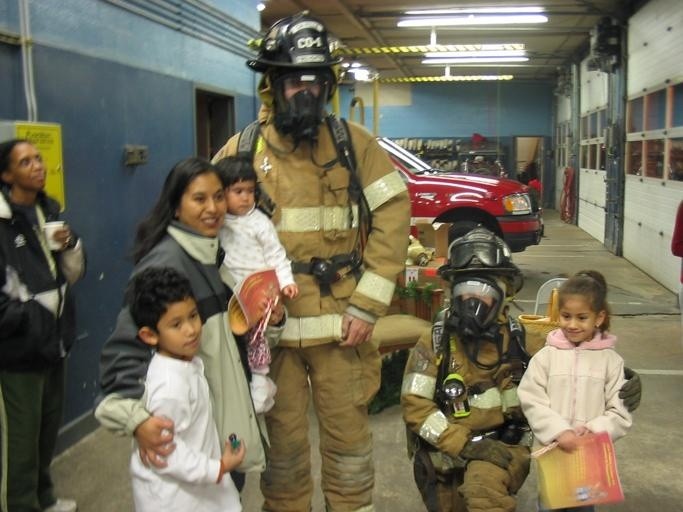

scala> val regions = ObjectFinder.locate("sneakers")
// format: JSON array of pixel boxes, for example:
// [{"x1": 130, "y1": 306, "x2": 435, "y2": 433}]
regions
[{"x1": 48, "y1": 499, "x2": 79, "y2": 512}]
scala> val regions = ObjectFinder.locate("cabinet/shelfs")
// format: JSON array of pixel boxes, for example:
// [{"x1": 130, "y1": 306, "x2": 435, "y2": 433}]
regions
[{"x1": 393, "y1": 136, "x2": 504, "y2": 176}]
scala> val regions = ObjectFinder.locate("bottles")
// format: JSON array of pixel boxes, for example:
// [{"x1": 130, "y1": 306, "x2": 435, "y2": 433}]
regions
[{"x1": 227, "y1": 433, "x2": 241, "y2": 453}]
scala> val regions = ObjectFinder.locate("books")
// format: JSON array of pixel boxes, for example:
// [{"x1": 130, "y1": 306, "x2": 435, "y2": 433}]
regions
[{"x1": 526, "y1": 431, "x2": 624, "y2": 510}]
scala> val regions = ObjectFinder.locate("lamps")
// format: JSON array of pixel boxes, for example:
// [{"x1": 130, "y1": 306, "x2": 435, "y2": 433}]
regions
[
  {"x1": 395, "y1": 12, "x2": 548, "y2": 27},
  {"x1": 422, "y1": 50, "x2": 531, "y2": 65}
]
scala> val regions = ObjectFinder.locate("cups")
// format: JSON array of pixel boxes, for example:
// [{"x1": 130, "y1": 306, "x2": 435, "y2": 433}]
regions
[{"x1": 43, "y1": 221, "x2": 66, "y2": 252}]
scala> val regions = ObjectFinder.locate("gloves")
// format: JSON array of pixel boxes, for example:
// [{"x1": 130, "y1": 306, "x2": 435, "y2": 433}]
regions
[
  {"x1": 618, "y1": 366, "x2": 642, "y2": 411},
  {"x1": 461, "y1": 436, "x2": 512, "y2": 469}
]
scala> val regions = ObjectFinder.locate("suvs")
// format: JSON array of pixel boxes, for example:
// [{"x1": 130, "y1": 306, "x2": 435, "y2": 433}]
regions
[{"x1": 370, "y1": 136, "x2": 544, "y2": 260}]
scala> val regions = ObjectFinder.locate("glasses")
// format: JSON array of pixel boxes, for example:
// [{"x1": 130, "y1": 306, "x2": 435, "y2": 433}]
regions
[{"x1": 449, "y1": 241, "x2": 503, "y2": 268}]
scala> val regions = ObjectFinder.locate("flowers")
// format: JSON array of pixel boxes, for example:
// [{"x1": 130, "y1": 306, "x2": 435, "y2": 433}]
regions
[{"x1": 526, "y1": 179, "x2": 542, "y2": 195}]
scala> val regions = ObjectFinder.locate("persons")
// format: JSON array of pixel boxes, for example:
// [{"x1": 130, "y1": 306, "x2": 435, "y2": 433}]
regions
[
  {"x1": 401, "y1": 227, "x2": 641, "y2": 512},
  {"x1": 514, "y1": 269, "x2": 632, "y2": 512},
  {"x1": 0, "y1": 139, "x2": 89, "y2": 512},
  {"x1": 209, "y1": 13, "x2": 412, "y2": 511},
  {"x1": 95, "y1": 156, "x2": 299, "y2": 510},
  {"x1": 671, "y1": 200, "x2": 682, "y2": 285}
]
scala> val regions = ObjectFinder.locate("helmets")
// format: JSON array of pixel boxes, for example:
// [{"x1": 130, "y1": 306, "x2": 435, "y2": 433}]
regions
[
  {"x1": 439, "y1": 227, "x2": 524, "y2": 296},
  {"x1": 247, "y1": 15, "x2": 342, "y2": 71}
]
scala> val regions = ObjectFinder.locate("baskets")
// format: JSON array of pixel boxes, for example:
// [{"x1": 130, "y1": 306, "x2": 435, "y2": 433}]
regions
[{"x1": 518, "y1": 289, "x2": 562, "y2": 357}]
